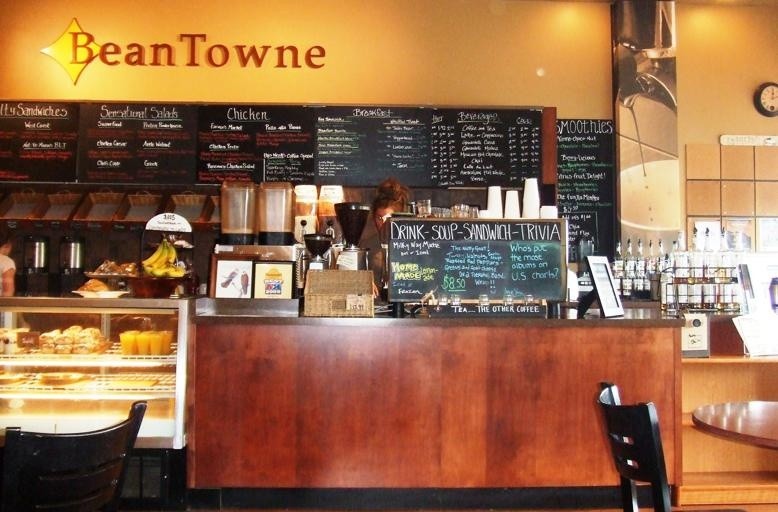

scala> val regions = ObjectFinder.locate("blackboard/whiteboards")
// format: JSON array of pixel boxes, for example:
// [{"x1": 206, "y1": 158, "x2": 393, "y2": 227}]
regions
[
  {"x1": 388, "y1": 217, "x2": 567, "y2": 302},
  {"x1": 317, "y1": 107, "x2": 433, "y2": 184},
  {"x1": 433, "y1": 109, "x2": 544, "y2": 185},
  {"x1": 76, "y1": 101, "x2": 198, "y2": 183},
  {"x1": 555, "y1": 118, "x2": 615, "y2": 264},
  {"x1": 0, "y1": 99, "x2": 80, "y2": 183},
  {"x1": 196, "y1": 104, "x2": 318, "y2": 187}
]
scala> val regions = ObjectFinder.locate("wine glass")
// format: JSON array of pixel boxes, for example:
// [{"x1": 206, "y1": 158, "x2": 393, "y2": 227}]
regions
[
  {"x1": 332, "y1": 201, "x2": 371, "y2": 251},
  {"x1": 303, "y1": 234, "x2": 333, "y2": 262}
]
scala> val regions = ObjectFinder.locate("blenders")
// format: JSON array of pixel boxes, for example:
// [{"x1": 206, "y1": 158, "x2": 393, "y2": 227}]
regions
[{"x1": 574, "y1": 230, "x2": 595, "y2": 285}]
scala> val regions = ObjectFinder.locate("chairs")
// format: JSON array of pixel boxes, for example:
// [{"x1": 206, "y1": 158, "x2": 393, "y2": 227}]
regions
[
  {"x1": 587, "y1": 380, "x2": 750, "y2": 512},
  {"x1": 0, "y1": 399, "x2": 148, "y2": 512}
]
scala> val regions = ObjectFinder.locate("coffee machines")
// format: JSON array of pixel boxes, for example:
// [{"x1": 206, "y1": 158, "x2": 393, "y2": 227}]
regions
[
  {"x1": 57, "y1": 235, "x2": 83, "y2": 296},
  {"x1": 21, "y1": 235, "x2": 49, "y2": 297}
]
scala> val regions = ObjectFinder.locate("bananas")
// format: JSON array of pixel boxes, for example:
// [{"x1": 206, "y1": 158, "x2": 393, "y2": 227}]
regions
[{"x1": 142, "y1": 238, "x2": 187, "y2": 279}]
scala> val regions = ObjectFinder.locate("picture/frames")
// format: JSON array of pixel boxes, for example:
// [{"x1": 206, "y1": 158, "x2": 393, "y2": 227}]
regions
[
  {"x1": 208, "y1": 253, "x2": 260, "y2": 298},
  {"x1": 584, "y1": 254, "x2": 623, "y2": 319},
  {"x1": 250, "y1": 260, "x2": 297, "y2": 299}
]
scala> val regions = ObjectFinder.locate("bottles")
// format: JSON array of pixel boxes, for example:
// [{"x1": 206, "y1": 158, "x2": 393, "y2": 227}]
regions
[{"x1": 614, "y1": 236, "x2": 738, "y2": 310}]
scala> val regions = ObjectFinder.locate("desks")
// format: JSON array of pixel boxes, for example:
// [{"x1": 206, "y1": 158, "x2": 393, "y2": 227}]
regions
[{"x1": 690, "y1": 397, "x2": 778, "y2": 451}]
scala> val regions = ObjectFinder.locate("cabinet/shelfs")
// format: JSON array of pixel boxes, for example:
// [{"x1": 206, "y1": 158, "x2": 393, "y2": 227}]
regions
[{"x1": 0, "y1": 295, "x2": 194, "y2": 452}]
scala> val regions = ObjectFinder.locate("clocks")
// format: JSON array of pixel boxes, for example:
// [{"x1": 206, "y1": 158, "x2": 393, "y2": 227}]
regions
[{"x1": 752, "y1": 81, "x2": 778, "y2": 117}]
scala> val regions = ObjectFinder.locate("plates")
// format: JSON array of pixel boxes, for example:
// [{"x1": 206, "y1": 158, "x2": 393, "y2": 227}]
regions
[
  {"x1": 83, "y1": 271, "x2": 137, "y2": 279},
  {"x1": 71, "y1": 290, "x2": 130, "y2": 298}
]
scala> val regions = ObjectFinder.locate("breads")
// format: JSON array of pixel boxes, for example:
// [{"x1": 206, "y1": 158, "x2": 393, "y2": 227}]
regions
[{"x1": 40, "y1": 324, "x2": 102, "y2": 354}]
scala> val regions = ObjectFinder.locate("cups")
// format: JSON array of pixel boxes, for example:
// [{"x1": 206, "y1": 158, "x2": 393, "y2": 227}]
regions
[
  {"x1": 392, "y1": 302, "x2": 404, "y2": 315},
  {"x1": 547, "y1": 302, "x2": 561, "y2": 316},
  {"x1": 407, "y1": 178, "x2": 559, "y2": 219},
  {"x1": 119, "y1": 328, "x2": 173, "y2": 355}
]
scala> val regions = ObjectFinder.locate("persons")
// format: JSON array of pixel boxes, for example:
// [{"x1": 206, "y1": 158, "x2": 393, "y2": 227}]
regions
[
  {"x1": 356, "y1": 177, "x2": 415, "y2": 306},
  {"x1": 0, "y1": 228, "x2": 18, "y2": 298}
]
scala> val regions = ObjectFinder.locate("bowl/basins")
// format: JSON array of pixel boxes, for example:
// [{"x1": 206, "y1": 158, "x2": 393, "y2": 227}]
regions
[{"x1": 123, "y1": 277, "x2": 188, "y2": 299}]
